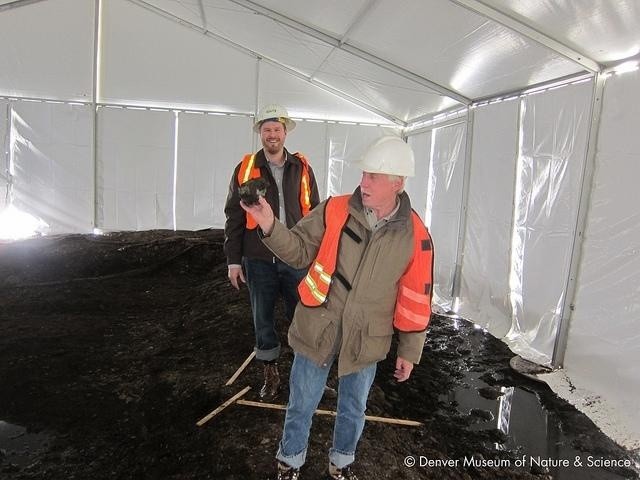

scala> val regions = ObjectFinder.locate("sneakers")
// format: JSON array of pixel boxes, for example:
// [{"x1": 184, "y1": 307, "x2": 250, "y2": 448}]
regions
[
  {"x1": 278, "y1": 463, "x2": 300, "y2": 479},
  {"x1": 328, "y1": 461, "x2": 358, "y2": 480}
]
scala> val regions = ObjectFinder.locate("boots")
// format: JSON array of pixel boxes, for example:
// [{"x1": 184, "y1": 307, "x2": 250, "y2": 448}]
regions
[
  {"x1": 322, "y1": 384, "x2": 338, "y2": 401},
  {"x1": 259, "y1": 363, "x2": 282, "y2": 403}
]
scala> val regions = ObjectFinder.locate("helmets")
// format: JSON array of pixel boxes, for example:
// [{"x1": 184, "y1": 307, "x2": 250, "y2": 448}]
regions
[
  {"x1": 253, "y1": 103, "x2": 297, "y2": 134},
  {"x1": 355, "y1": 134, "x2": 417, "y2": 178}
]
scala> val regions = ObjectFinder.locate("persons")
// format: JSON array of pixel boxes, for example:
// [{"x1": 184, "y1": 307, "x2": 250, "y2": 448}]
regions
[
  {"x1": 236, "y1": 132, "x2": 436, "y2": 480},
  {"x1": 224, "y1": 100, "x2": 339, "y2": 401}
]
